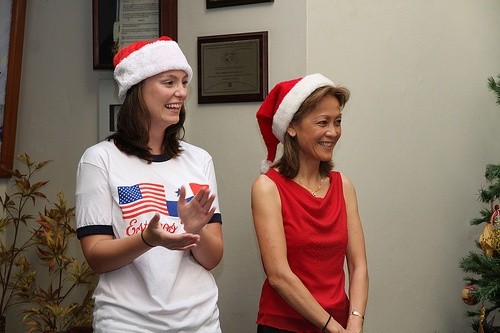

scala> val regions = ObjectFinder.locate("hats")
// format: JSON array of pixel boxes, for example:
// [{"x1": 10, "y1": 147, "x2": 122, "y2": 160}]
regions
[
  {"x1": 113, "y1": 36, "x2": 192, "y2": 99},
  {"x1": 255, "y1": 74, "x2": 337, "y2": 175}
]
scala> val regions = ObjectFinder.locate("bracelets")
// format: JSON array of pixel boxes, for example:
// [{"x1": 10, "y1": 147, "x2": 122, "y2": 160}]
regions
[
  {"x1": 140, "y1": 228, "x2": 156, "y2": 248},
  {"x1": 348, "y1": 310, "x2": 365, "y2": 326},
  {"x1": 323, "y1": 315, "x2": 333, "y2": 332}
]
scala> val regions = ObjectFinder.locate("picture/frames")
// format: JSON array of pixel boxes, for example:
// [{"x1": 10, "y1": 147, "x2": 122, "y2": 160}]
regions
[{"x1": 0, "y1": 0, "x2": 25, "y2": 177}]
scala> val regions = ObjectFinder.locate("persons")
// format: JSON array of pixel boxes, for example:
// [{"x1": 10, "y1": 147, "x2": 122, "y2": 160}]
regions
[
  {"x1": 250, "y1": 73, "x2": 370, "y2": 333},
  {"x1": 74, "y1": 36, "x2": 225, "y2": 333}
]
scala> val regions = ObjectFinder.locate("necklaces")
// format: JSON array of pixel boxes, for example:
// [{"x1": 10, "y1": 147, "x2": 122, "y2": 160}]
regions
[{"x1": 295, "y1": 175, "x2": 324, "y2": 197}]
